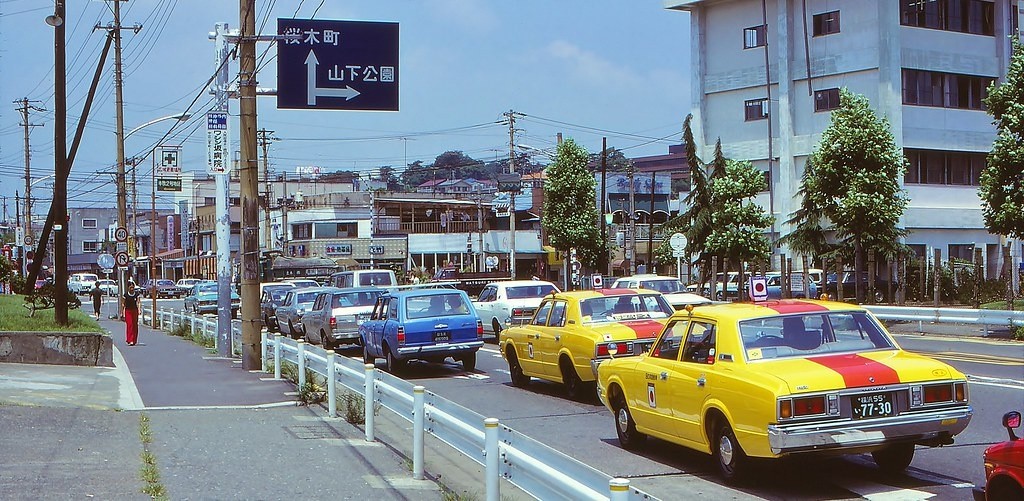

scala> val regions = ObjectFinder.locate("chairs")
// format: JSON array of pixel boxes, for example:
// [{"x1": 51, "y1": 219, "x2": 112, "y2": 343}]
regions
[
  {"x1": 782, "y1": 317, "x2": 821, "y2": 349},
  {"x1": 429, "y1": 297, "x2": 445, "y2": 315},
  {"x1": 615, "y1": 302, "x2": 633, "y2": 313},
  {"x1": 580, "y1": 302, "x2": 593, "y2": 316}
]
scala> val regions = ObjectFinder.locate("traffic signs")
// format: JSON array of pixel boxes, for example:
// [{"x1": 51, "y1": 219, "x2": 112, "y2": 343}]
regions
[{"x1": 276, "y1": 16, "x2": 399, "y2": 112}]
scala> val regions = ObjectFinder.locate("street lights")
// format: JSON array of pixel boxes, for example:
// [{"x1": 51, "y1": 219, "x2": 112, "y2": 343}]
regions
[
  {"x1": 26, "y1": 173, "x2": 55, "y2": 278},
  {"x1": 518, "y1": 133, "x2": 573, "y2": 292},
  {"x1": 116, "y1": 111, "x2": 192, "y2": 320}
]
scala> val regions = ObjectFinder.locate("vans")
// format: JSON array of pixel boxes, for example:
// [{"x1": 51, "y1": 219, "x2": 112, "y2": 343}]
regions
[
  {"x1": 328, "y1": 269, "x2": 400, "y2": 294},
  {"x1": 71, "y1": 274, "x2": 99, "y2": 296}
]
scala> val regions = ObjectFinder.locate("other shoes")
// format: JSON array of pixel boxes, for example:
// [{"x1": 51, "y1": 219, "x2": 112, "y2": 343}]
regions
[{"x1": 128, "y1": 342, "x2": 133, "y2": 346}]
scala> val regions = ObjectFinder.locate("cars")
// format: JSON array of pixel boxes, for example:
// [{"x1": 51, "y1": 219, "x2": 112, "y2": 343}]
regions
[
  {"x1": 275, "y1": 286, "x2": 352, "y2": 340},
  {"x1": 499, "y1": 274, "x2": 708, "y2": 402},
  {"x1": 184, "y1": 282, "x2": 241, "y2": 319},
  {"x1": 471, "y1": 280, "x2": 565, "y2": 345},
  {"x1": 597, "y1": 275, "x2": 972, "y2": 488},
  {"x1": 91, "y1": 279, "x2": 115, "y2": 295},
  {"x1": 357, "y1": 281, "x2": 485, "y2": 372},
  {"x1": 811, "y1": 270, "x2": 899, "y2": 303},
  {"x1": 685, "y1": 268, "x2": 827, "y2": 299},
  {"x1": 146, "y1": 279, "x2": 181, "y2": 299},
  {"x1": 191, "y1": 279, "x2": 218, "y2": 296},
  {"x1": 260, "y1": 278, "x2": 320, "y2": 333},
  {"x1": 605, "y1": 274, "x2": 711, "y2": 311},
  {"x1": 744, "y1": 273, "x2": 817, "y2": 301},
  {"x1": 301, "y1": 283, "x2": 386, "y2": 352},
  {"x1": 35, "y1": 280, "x2": 44, "y2": 289},
  {"x1": 109, "y1": 279, "x2": 136, "y2": 297},
  {"x1": 175, "y1": 278, "x2": 200, "y2": 297},
  {"x1": 972, "y1": 411, "x2": 1024, "y2": 501}
]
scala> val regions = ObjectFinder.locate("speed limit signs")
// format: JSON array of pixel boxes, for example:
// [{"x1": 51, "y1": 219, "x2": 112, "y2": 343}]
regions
[{"x1": 115, "y1": 227, "x2": 128, "y2": 243}]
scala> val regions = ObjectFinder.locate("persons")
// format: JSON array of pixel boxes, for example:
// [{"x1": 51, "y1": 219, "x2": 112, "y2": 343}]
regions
[
  {"x1": 358, "y1": 292, "x2": 369, "y2": 306},
  {"x1": 89, "y1": 281, "x2": 105, "y2": 320},
  {"x1": 413, "y1": 275, "x2": 420, "y2": 284},
  {"x1": 122, "y1": 281, "x2": 141, "y2": 346},
  {"x1": 447, "y1": 296, "x2": 463, "y2": 314}
]
